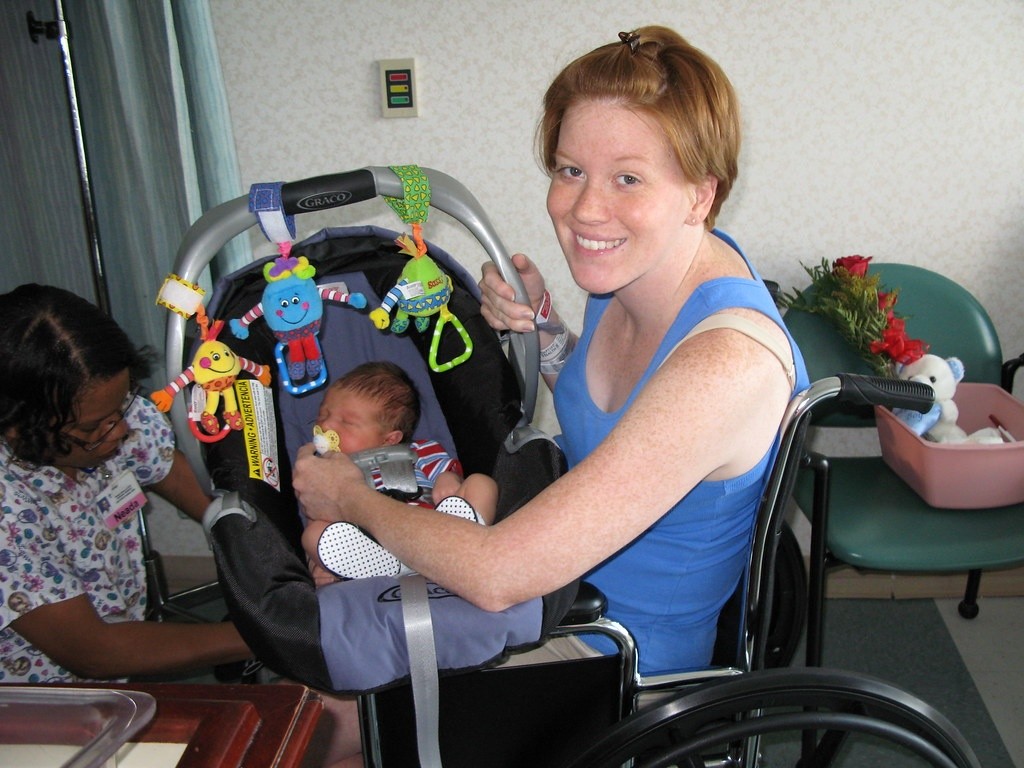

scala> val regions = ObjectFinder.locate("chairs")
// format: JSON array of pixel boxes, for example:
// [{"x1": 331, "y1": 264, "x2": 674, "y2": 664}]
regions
[{"x1": 782, "y1": 264, "x2": 1024, "y2": 768}]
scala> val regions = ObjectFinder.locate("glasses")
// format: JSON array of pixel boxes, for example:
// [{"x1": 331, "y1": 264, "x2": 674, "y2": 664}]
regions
[{"x1": 52, "y1": 381, "x2": 142, "y2": 452}]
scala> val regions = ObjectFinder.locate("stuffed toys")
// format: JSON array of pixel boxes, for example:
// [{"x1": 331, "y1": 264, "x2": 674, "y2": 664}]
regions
[
  {"x1": 891, "y1": 355, "x2": 969, "y2": 444},
  {"x1": 369, "y1": 224, "x2": 474, "y2": 372},
  {"x1": 149, "y1": 302, "x2": 271, "y2": 443},
  {"x1": 229, "y1": 242, "x2": 366, "y2": 396}
]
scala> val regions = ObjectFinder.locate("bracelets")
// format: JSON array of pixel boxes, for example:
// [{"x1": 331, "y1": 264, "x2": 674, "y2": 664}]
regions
[{"x1": 535, "y1": 288, "x2": 576, "y2": 376}]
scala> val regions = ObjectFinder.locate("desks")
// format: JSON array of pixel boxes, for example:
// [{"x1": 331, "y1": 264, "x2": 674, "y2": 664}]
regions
[{"x1": 0, "y1": 684, "x2": 325, "y2": 768}]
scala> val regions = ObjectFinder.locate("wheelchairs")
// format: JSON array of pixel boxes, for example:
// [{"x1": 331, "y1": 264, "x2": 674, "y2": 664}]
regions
[{"x1": 331, "y1": 279, "x2": 980, "y2": 768}]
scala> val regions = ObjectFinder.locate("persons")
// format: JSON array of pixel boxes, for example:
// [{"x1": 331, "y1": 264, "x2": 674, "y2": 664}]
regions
[
  {"x1": 99, "y1": 499, "x2": 111, "y2": 515},
  {"x1": 1, "y1": 280, "x2": 259, "y2": 684},
  {"x1": 274, "y1": 25, "x2": 813, "y2": 768},
  {"x1": 294, "y1": 358, "x2": 500, "y2": 596}
]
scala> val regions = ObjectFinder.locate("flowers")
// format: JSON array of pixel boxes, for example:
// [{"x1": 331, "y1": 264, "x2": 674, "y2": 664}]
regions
[{"x1": 775, "y1": 256, "x2": 930, "y2": 380}]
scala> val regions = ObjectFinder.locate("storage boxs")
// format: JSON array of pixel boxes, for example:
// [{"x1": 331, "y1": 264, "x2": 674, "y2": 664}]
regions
[{"x1": 871, "y1": 381, "x2": 1024, "y2": 510}]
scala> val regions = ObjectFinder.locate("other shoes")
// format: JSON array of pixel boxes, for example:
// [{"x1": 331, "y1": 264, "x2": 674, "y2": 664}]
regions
[
  {"x1": 434, "y1": 495, "x2": 479, "y2": 524},
  {"x1": 317, "y1": 521, "x2": 401, "y2": 580}
]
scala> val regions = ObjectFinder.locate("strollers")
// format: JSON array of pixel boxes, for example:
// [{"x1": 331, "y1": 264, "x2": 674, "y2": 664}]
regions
[{"x1": 161, "y1": 165, "x2": 588, "y2": 689}]
